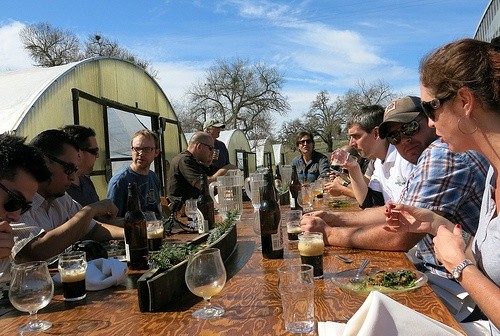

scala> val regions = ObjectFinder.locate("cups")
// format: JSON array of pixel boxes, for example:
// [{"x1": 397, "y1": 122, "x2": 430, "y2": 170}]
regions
[
  {"x1": 298, "y1": 232, "x2": 324, "y2": 279},
  {"x1": 209, "y1": 176, "x2": 243, "y2": 221},
  {"x1": 146, "y1": 221, "x2": 163, "y2": 253},
  {"x1": 277, "y1": 264, "x2": 315, "y2": 332},
  {"x1": 285, "y1": 210, "x2": 302, "y2": 242},
  {"x1": 330, "y1": 149, "x2": 349, "y2": 172},
  {"x1": 58, "y1": 251, "x2": 87, "y2": 302},
  {"x1": 244, "y1": 173, "x2": 264, "y2": 209}
]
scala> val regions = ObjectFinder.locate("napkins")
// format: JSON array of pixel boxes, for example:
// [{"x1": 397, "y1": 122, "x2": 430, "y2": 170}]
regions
[{"x1": 318, "y1": 291, "x2": 464, "y2": 336}]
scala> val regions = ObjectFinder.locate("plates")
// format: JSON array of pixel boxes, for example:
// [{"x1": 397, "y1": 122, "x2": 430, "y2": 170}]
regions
[{"x1": 331, "y1": 266, "x2": 428, "y2": 293}]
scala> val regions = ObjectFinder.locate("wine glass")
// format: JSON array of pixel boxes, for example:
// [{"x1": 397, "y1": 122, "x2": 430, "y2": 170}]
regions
[
  {"x1": 298, "y1": 191, "x2": 313, "y2": 213},
  {"x1": 311, "y1": 183, "x2": 321, "y2": 202},
  {"x1": 144, "y1": 211, "x2": 157, "y2": 224},
  {"x1": 8, "y1": 262, "x2": 54, "y2": 333},
  {"x1": 185, "y1": 199, "x2": 198, "y2": 231},
  {"x1": 185, "y1": 248, "x2": 226, "y2": 317}
]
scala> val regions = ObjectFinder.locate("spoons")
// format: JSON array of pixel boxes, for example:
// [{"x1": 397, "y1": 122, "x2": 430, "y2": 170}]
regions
[{"x1": 335, "y1": 255, "x2": 388, "y2": 263}]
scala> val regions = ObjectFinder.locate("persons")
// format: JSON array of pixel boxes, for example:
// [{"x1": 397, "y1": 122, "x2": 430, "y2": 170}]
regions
[
  {"x1": 167, "y1": 118, "x2": 231, "y2": 205},
  {"x1": 300, "y1": 96, "x2": 491, "y2": 280},
  {"x1": 107, "y1": 129, "x2": 161, "y2": 222},
  {"x1": 329, "y1": 104, "x2": 417, "y2": 210},
  {"x1": 8, "y1": 129, "x2": 124, "y2": 265},
  {"x1": 58, "y1": 125, "x2": 108, "y2": 223},
  {"x1": 0, "y1": 131, "x2": 54, "y2": 282},
  {"x1": 319, "y1": 144, "x2": 369, "y2": 182},
  {"x1": 292, "y1": 132, "x2": 331, "y2": 184},
  {"x1": 381, "y1": 38, "x2": 500, "y2": 336}
]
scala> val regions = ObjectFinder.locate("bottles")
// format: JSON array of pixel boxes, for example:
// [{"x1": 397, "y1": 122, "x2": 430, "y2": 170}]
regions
[
  {"x1": 259, "y1": 172, "x2": 284, "y2": 258},
  {"x1": 274, "y1": 165, "x2": 282, "y2": 182},
  {"x1": 197, "y1": 174, "x2": 214, "y2": 234},
  {"x1": 289, "y1": 165, "x2": 301, "y2": 210},
  {"x1": 124, "y1": 183, "x2": 148, "y2": 269}
]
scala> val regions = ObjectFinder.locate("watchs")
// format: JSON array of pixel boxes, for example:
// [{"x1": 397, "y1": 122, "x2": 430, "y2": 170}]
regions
[{"x1": 451, "y1": 259, "x2": 475, "y2": 282}]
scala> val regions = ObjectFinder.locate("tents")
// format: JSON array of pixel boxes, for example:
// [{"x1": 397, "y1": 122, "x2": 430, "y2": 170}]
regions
[
  {"x1": 248, "y1": 138, "x2": 275, "y2": 168},
  {"x1": 0, "y1": 56, "x2": 188, "y2": 200},
  {"x1": 183, "y1": 129, "x2": 257, "y2": 183},
  {"x1": 272, "y1": 143, "x2": 287, "y2": 168}
]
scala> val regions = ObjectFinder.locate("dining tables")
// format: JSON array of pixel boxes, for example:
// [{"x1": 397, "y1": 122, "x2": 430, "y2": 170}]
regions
[{"x1": 0, "y1": 184, "x2": 470, "y2": 336}]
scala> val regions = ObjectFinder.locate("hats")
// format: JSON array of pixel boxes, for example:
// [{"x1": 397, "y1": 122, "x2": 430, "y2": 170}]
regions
[
  {"x1": 378, "y1": 96, "x2": 421, "y2": 140},
  {"x1": 203, "y1": 119, "x2": 225, "y2": 130}
]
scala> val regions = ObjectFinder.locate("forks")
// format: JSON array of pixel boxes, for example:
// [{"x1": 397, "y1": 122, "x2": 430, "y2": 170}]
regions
[{"x1": 348, "y1": 259, "x2": 370, "y2": 282}]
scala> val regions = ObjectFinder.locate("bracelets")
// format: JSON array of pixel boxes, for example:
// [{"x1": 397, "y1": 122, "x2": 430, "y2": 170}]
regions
[
  {"x1": 462, "y1": 229, "x2": 472, "y2": 253},
  {"x1": 343, "y1": 181, "x2": 350, "y2": 186}
]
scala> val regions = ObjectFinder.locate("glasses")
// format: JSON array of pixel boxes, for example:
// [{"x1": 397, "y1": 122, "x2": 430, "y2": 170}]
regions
[
  {"x1": 201, "y1": 143, "x2": 216, "y2": 150},
  {"x1": 132, "y1": 146, "x2": 155, "y2": 153},
  {"x1": 386, "y1": 116, "x2": 426, "y2": 145},
  {"x1": 420, "y1": 91, "x2": 455, "y2": 123},
  {"x1": 80, "y1": 147, "x2": 99, "y2": 154},
  {"x1": 45, "y1": 154, "x2": 79, "y2": 175},
  {"x1": 0, "y1": 183, "x2": 32, "y2": 215},
  {"x1": 298, "y1": 139, "x2": 313, "y2": 144}
]
